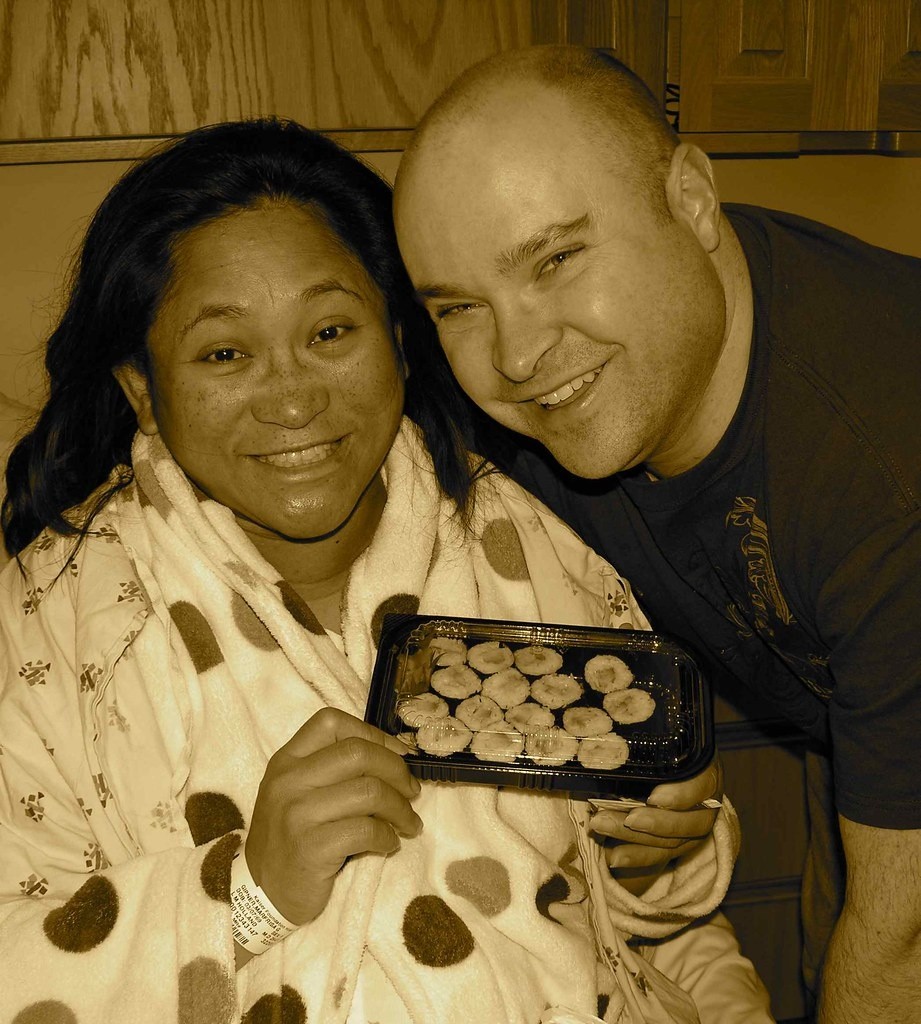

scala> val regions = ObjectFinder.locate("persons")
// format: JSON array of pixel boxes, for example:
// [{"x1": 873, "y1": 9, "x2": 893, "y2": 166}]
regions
[
  {"x1": 0, "y1": 44, "x2": 740, "y2": 1024},
  {"x1": 392, "y1": 45, "x2": 921, "y2": 1024}
]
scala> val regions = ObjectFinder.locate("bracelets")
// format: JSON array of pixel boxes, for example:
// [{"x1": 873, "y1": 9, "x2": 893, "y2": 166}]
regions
[{"x1": 229, "y1": 844, "x2": 296, "y2": 955}]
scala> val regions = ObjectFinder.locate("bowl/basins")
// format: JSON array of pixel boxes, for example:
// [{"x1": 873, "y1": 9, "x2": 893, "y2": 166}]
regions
[{"x1": 360, "y1": 613, "x2": 714, "y2": 800}]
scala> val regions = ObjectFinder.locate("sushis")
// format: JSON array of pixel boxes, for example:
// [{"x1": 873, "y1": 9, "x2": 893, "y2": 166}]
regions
[{"x1": 396, "y1": 637, "x2": 659, "y2": 773}]
